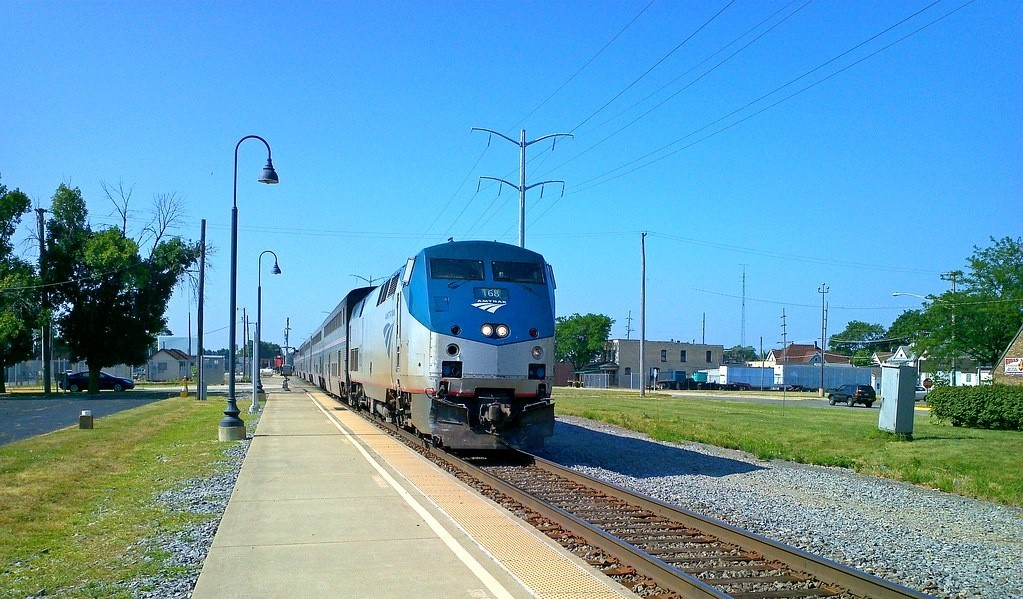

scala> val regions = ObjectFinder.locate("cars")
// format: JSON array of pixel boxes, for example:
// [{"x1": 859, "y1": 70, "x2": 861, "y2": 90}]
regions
[
  {"x1": 59, "y1": 370, "x2": 136, "y2": 392},
  {"x1": 914, "y1": 386, "x2": 931, "y2": 402}
]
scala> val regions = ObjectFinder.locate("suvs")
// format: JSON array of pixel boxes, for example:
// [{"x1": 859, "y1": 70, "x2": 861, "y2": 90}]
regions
[{"x1": 827, "y1": 383, "x2": 876, "y2": 408}]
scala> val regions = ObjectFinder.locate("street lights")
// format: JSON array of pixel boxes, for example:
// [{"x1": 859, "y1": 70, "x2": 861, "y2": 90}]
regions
[
  {"x1": 237, "y1": 307, "x2": 246, "y2": 377},
  {"x1": 240, "y1": 315, "x2": 251, "y2": 378},
  {"x1": 218, "y1": 132, "x2": 281, "y2": 441},
  {"x1": 255, "y1": 249, "x2": 283, "y2": 402},
  {"x1": 891, "y1": 292, "x2": 956, "y2": 386}
]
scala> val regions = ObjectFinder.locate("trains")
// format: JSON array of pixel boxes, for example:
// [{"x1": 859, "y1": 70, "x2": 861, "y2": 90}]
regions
[{"x1": 291, "y1": 236, "x2": 557, "y2": 452}]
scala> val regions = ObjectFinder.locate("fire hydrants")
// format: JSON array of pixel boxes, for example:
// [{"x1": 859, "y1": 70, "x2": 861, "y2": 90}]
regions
[{"x1": 181, "y1": 375, "x2": 189, "y2": 392}]
